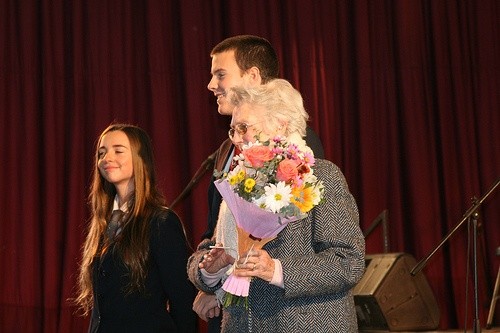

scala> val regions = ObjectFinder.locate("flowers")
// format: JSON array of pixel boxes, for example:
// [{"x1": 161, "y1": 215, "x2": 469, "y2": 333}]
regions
[{"x1": 212, "y1": 131, "x2": 327, "y2": 311}]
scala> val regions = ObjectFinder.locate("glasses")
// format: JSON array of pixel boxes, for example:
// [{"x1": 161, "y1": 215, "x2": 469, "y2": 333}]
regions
[{"x1": 228, "y1": 120, "x2": 265, "y2": 140}]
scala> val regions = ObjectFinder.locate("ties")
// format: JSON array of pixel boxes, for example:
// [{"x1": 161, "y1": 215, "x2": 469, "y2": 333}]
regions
[
  {"x1": 230, "y1": 145, "x2": 244, "y2": 172},
  {"x1": 102, "y1": 209, "x2": 126, "y2": 249}
]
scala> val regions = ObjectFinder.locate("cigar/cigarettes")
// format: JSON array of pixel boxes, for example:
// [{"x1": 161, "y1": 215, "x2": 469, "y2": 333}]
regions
[{"x1": 209, "y1": 246, "x2": 230, "y2": 249}]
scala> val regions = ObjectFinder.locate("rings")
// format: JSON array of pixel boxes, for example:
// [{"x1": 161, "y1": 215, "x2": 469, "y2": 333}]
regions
[{"x1": 252, "y1": 263, "x2": 256, "y2": 270}]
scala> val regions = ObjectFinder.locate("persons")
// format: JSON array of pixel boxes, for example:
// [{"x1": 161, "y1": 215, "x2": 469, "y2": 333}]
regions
[
  {"x1": 186, "y1": 78, "x2": 365, "y2": 333},
  {"x1": 75, "y1": 124, "x2": 195, "y2": 333},
  {"x1": 195, "y1": 36, "x2": 323, "y2": 332}
]
